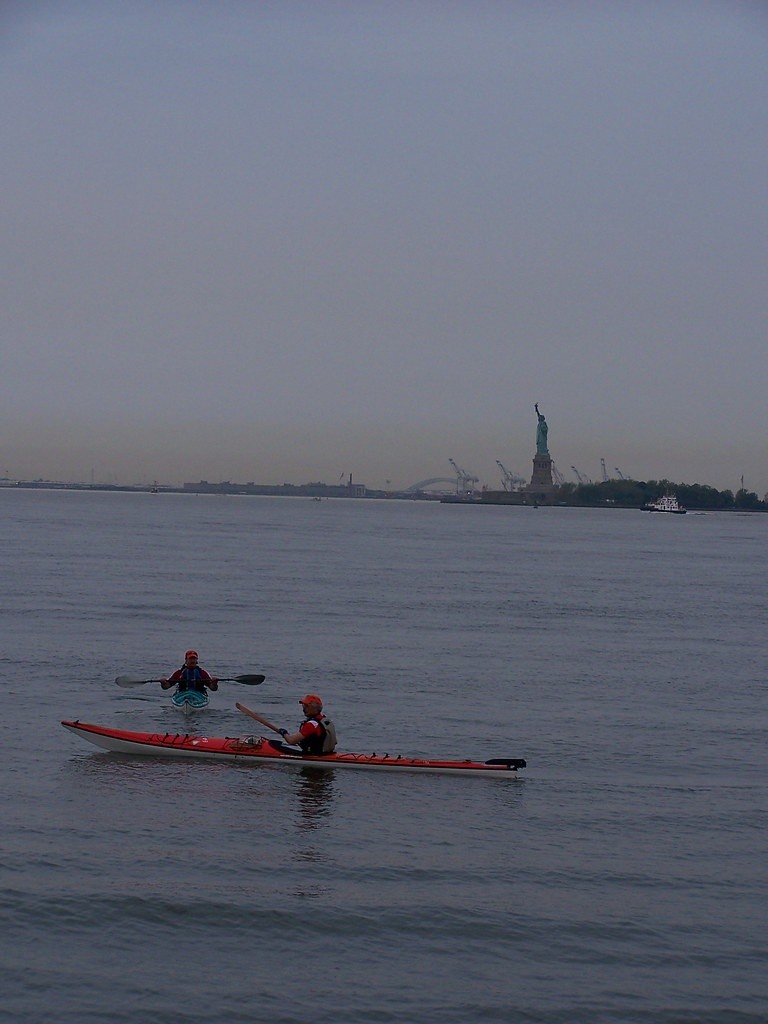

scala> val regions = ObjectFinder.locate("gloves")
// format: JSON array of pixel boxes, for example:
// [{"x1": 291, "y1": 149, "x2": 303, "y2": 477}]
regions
[{"x1": 278, "y1": 728, "x2": 288, "y2": 737}]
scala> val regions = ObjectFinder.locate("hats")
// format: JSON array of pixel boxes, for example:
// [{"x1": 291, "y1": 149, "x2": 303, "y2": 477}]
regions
[
  {"x1": 298, "y1": 695, "x2": 322, "y2": 707},
  {"x1": 185, "y1": 650, "x2": 199, "y2": 659}
]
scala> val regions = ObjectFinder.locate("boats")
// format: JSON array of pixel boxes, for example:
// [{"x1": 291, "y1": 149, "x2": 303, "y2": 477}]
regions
[
  {"x1": 59, "y1": 719, "x2": 528, "y2": 778},
  {"x1": 172, "y1": 688, "x2": 213, "y2": 715},
  {"x1": 639, "y1": 492, "x2": 688, "y2": 514}
]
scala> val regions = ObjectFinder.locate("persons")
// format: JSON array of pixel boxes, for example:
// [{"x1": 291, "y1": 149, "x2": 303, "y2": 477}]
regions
[
  {"x1": 534, "y1": 403, "x2": 549, "y2": 454},
  {"x1": 278, "y1": 695, "x2": 337, "y2": 754},
  {"x1": 160, "y1": 650, "x2": 219, "y2": 697}
]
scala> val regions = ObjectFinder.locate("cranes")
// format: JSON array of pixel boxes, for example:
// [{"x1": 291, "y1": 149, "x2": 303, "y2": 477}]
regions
[
  {"x1": 569, "y1": 464, "x2": 587, "y2": 485},
  {"x1": 447, "y1": 456, "x2": 480, "y2": 497},
  {"x1": 615, "y1": 465, "x2": 628, "y2": 481},
  {"x1": 549, "y1": 463, "x2": 567, "y2": 486},
  {"x1": 497, "y1": 460, "x2": 518, "y2": 494},
  {"x1": 599, "y1": 457, "x2": 610, "y2": 484}
]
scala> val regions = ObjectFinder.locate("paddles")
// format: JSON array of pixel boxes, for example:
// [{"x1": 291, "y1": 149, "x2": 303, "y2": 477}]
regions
[
  {"x1": 115, "y1": 670, "x2": 267, "y2": 688},
  {"x1": 234, "y1": 702, "x2": 288, "y2": 740}
]
